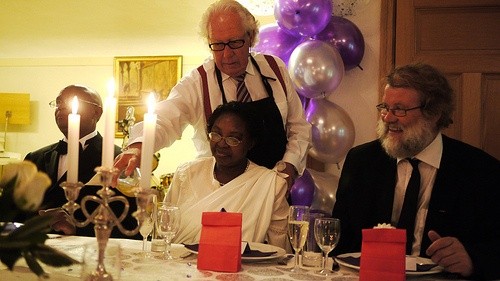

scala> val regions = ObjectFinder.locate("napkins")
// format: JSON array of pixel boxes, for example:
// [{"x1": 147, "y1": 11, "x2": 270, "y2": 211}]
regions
[
  {"x1": 334, "y1": 255, "x2": 439, "y2": 273},
  {"x1": 180, "y1": 240, "x2": 278, "y2": 257}
]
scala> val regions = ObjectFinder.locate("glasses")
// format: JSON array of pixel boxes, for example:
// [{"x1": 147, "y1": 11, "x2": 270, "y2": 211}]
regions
[
  {"x1": 206, "y1": 131, "x2": 246, "y2": 147},
  {"x1": 376, "y1": 103, "x2": 426, "y2": 117},
  {"x1": 208, "y1": 34, "x2": 247, "y2": 51},
  {"x1": 49, "y1": 96, "x2": 102, "y2": 109}
]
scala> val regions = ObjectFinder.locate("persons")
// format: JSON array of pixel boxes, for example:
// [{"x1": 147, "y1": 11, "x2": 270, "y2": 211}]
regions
[
  {"x1": 113, "y1": 0, "x2": 313, "y2": 207},
  {"x1": 158, "y1": 100, "x2": 294, "y2": 254},
  {"x1": 0, "y1": 84, "x2": 152, "y2": 240},
  {"x1": 319, "y1": 61, "x2": 500, "y2": 281}
]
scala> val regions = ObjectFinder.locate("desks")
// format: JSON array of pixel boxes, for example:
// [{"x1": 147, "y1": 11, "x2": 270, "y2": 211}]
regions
[{"x1": 0, "y1": 234, "x2": 470, "y2": 281}]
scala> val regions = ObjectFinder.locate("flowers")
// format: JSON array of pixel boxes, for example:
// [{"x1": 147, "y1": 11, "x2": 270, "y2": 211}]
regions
[{"x1": 0, "y1": 159, "x2": 84, "y2": 277}]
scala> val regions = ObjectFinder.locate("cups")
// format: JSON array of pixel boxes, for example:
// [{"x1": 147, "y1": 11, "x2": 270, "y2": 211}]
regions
[
  {"x1": 151, "y1": 201, "x2": 171, "y2": 252},
  {"x1": 303, "y1": 212, "x2": 324, "y2": 268},
  {"x1": 81, "y1": 240, "x2": 123, "y2": 281}
]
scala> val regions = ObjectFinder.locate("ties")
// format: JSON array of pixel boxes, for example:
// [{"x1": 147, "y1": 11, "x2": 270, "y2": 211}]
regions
[
  {"x1": 232, "y1": 72, "x2": 252, "y2": 103},
  {"x1": 397, "y1": 158, "x2": 422, "y2": 256}
]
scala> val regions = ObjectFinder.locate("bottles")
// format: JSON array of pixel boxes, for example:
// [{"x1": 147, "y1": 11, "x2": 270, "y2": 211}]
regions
[{"x1": 113, "y1": 165, "x2": 143, "y2": 197}]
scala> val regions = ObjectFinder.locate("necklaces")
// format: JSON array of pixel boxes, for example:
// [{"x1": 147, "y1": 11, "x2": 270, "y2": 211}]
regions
[{"x1": 212, "y1": 161, "x2": 249, "y2": 186}]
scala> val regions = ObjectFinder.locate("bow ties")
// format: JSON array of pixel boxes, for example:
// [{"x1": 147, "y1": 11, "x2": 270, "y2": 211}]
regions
[{"x1": 56, "y1": 139, "x2": 83, "y2": 155}]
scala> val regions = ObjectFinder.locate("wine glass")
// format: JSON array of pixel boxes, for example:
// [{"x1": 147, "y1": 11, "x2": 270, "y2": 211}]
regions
[
  {"x1": 136, "y1": 193, "x2": 158, "y2": 257},
  {"x1": 312, "y1": 217, "x2": 341, "y2": 278},
  {"x1": 157, "y1": 206, "x2": 179, "y2": 262},
  {"x1": 287, "y1": 205, "x2": 311, "y2": 276}
]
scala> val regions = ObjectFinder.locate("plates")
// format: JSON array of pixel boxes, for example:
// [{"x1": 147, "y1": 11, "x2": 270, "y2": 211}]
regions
[
  {"x1": 182, "y1": 240, "x2": 286, "y2": 262},
  {"x1": 334, "y1": 252, "x2": 443, "y2": 276}
]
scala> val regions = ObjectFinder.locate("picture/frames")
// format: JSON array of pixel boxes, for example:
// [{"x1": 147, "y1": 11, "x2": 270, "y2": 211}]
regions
[{"x1": 113, "y1": 56, "x2": 183, "y2": 139}]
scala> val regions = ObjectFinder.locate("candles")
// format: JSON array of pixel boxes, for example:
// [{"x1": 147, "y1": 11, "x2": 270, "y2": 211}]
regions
[
  {"x1": 140, "y1": 93, "x2": 157, "y2": 192},
  {"x1": 101, "y1": 75, "x2": 117, "y2": 171},
  {"x1": 67, "y1": 96, "x2": 81, "y2": 183}
]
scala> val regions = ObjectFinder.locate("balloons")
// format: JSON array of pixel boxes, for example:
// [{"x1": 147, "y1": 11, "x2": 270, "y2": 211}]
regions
[{"x1": 249, "y1": 0, "x2": 366, "y2": 210}]
